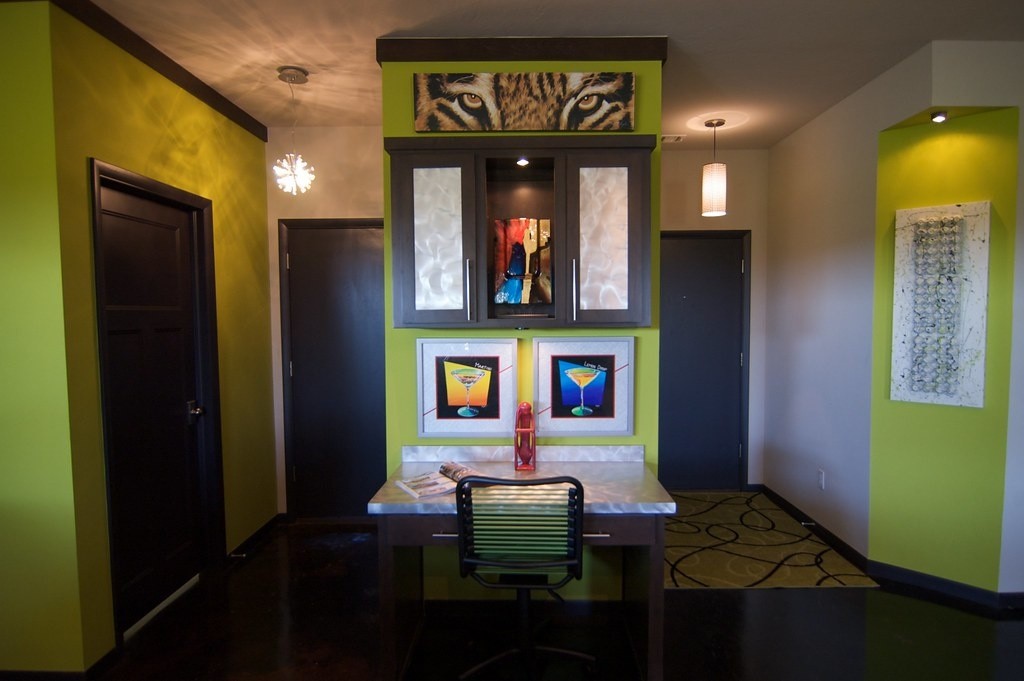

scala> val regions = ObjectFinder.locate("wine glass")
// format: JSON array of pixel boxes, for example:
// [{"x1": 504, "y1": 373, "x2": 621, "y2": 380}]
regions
[
  {"x1": 565, "y1": 368, "x2": 601, "y2": 416},
  {"x1": 452, "y1": 369, "x2": 486, "y2": 417}
]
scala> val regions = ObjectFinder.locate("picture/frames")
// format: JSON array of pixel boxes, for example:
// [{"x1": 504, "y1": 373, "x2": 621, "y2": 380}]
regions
[
  {"x1": 416, "y1": 338, "x2": 518, "y2": 438},
  {"x1": 532, "y1": 335, "x2": 636, "y2": 438}
]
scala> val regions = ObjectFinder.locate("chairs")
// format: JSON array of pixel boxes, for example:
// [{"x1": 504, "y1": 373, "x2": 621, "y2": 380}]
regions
[{"x1": 455, "y1": 474, "x2": 608, "y2": 681}]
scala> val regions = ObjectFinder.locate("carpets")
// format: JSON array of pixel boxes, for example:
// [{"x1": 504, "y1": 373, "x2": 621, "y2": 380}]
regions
[
  {"x1": 659, "y1": 490, "x2": 884, "y2": 590},
  {"x1": 104, "y1": 524, "x2": 380, "y2": 681}
]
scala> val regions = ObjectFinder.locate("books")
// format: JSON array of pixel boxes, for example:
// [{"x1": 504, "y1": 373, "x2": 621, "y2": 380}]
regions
[{"x1": 395, "y1": 461, "x2": 480, "y2": 500}]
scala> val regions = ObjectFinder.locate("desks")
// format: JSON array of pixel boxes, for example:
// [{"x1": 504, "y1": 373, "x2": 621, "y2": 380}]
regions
[{"x1": 367, "y1": 442, "x2": 677, "y2": 681}]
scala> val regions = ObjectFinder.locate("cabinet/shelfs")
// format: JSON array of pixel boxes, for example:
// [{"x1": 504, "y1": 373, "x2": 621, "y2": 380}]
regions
[{"x1": 383, "y1": 132, "x2": 657, "y2": 330}]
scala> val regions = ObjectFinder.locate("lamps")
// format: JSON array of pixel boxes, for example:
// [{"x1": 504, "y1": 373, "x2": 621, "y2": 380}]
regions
[
  {"x1": 273, "y1": 68, "x2": 316, "y2": 194},
  {"x1": 700, "y1": 118, "x2": 728, "y2": 218},
  {"x1": 516, "y1": 158, "x2": 530, "y2": 167},
  {"x1": 931, "y1": 111, "x2": 947, "y2": 123}
]
